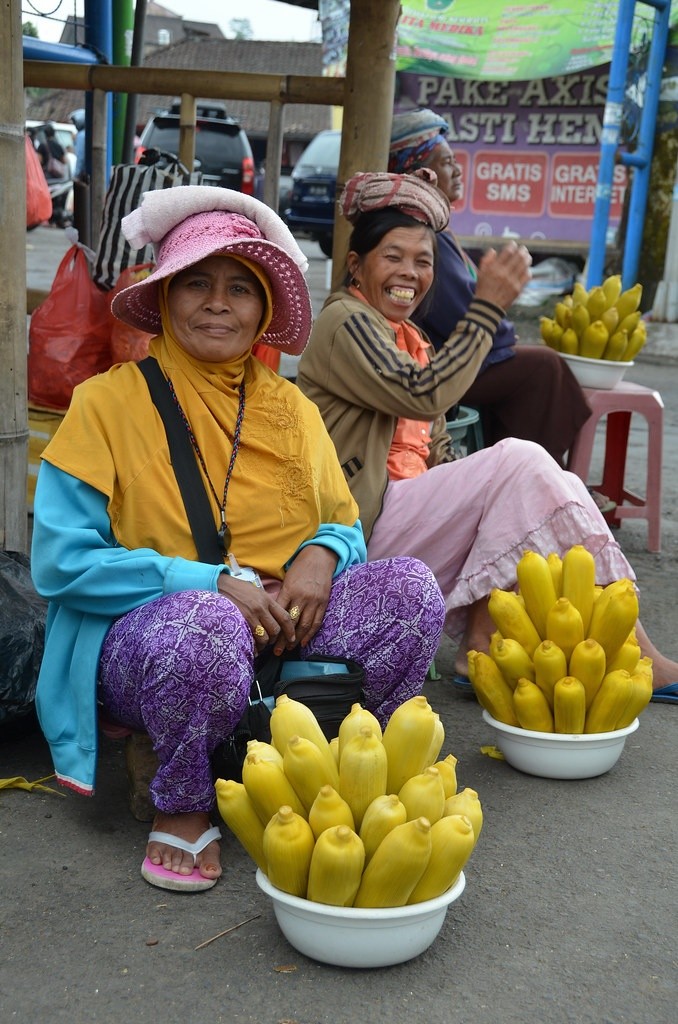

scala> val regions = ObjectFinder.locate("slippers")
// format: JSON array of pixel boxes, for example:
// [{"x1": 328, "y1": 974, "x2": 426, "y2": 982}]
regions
[
  {"x1": 139, "y1": 822, "x2": 223, "y2": 892},
  {"x1": 453, "y1": 675, "x2": 474, "y2": 693},
  {"x1": 651, "y1": 682, "x2": 677, "y2": 704}
]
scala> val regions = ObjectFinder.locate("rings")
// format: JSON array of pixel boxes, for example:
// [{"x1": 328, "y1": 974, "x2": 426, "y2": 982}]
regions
[
  {"x1": 252, "y1": 625, "x2": 266, "y2": 637},
  {"x1": 288, "y1": 605, "x2": 300, "y2": 620}
]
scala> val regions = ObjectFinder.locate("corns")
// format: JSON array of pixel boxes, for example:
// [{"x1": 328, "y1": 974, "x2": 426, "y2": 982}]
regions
[
  {"x1": 214, "y1": 694, "x2": 482, "y2": 909},
  {"x1": 540, "y1": 274, "x2": 649, "y2": 363},
  {"x1": 467, "y1": 542, "x2": 654, "y2": 736}
]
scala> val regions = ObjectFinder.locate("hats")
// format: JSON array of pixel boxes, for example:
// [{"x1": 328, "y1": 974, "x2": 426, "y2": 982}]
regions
[{"x1": 111, "y1": 209, "x2": 315, "y2": 356}]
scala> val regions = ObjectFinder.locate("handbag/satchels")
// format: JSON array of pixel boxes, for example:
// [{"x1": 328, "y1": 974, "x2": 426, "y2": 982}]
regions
[
  {"x1": 210, "y1": 655, "x2": 367, "y2": 785},
  {"x1": 26, "y1": 131, "x2": 205, "y2": 408}
]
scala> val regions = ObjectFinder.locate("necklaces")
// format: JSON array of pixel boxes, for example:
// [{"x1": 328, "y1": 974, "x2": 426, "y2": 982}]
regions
[{"x1": 165, "y1": 370, "x2": 246, "y2": 560}]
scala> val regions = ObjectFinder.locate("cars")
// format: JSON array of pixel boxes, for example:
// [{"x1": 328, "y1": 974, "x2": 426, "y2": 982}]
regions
[{"x1": 285, "y1": 130, "x2": 342, "y2": 258}]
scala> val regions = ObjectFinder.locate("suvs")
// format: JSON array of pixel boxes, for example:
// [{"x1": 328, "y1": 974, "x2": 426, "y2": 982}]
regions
[{"x1": 26, "y1": 96, "x2": 294, "y2": 225}]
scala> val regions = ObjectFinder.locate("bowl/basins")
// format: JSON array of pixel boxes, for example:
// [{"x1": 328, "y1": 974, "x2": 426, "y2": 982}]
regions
[
  {"x1": 255, "y1": 866, "x2": 467, "y2": 968},
  {"x1": 556, "y1": 352, "x2": 634, "y2": 390},
  {"x1": 481, "y1": 707, "x2": 639, "y2": 780}
]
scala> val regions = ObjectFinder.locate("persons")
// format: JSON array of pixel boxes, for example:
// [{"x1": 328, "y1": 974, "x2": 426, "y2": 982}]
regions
[
  {"x1": 388, "y1": 106, "x2": 619, "y2": 526},
  {"x1": 24, "y1": 108, "x2": 87, "y2": 230},
  {"x1": 297, "y1": 172, "x2": 677, "y2": 705},
  {"x1": 33, "y1": 184, "x2": 447, "y2": 891}
]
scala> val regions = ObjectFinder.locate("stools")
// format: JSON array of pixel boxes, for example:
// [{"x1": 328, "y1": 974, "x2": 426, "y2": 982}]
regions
[
  {"x1": 442, "y1": 405, "x2": 485, "y2": 459},
  {"x1": 562, "y1": 380, "x2": 665, "y2": 554}
]
